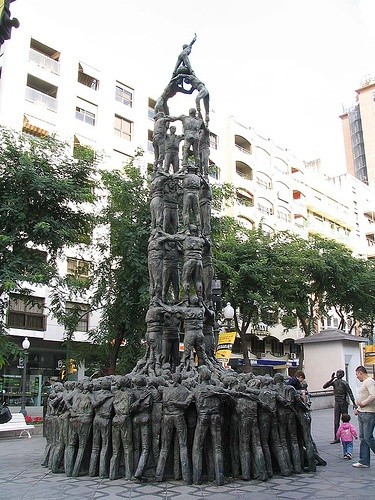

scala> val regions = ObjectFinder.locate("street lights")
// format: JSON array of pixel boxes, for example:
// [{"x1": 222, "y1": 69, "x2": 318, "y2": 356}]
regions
[
  {"x1": 223, "y1": 302, "x2": 235, "y2": 332},
  {"x1": 19, "y1": 337, "x2": 31, "y2": 417}
]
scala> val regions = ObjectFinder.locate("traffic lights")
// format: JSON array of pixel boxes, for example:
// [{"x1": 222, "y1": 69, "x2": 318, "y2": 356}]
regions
[
  {"x1": 69, "y1": 359, "x2": 78, "y2": 374},
  {"x1": 60, "y1": 370, "x2": 67, "y2": 381}
]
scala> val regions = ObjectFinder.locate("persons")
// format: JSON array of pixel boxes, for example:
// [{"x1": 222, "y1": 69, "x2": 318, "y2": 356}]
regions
[
  {"x1": 153, "y1": 107, "x2": 210, "y2": 181},
  {"x1": 144, "y1": 296, "x2": 216, "y2": 366},
  {"x1": 172, "y1": 33, "x2": 197, "y2": 75},
  {"x1": 336, "y1": 414, "x2": 357, "y2": 459},
  {"x1": 288, "y1": 370, "x2": 306, "y2": 391},
  {"x1": 323, "y1": 369, "x2": 358, "y2": 444},
  {"x1": 148, "y1": 224, "x2": 214, "y2": 301},
  {"x1": 150, "y1": 165, "x2": 211, "y2": 234},
  {"x1": 352, "y1": 366, "x2": 375, "y2": 467},
  {"x1": 154, "y1": 74, "x2": 210, "y2": 120},
  {"x1": 40, "y1": 356, "x2": 327, "y2": 484}
]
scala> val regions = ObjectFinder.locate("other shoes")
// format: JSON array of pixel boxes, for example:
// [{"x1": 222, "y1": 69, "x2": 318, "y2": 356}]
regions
[
  {"x1": 343, "y1": 455, "x2": 347, "y2": 459},
  {"x1": 352, "y1": 462, "x2": 369, "y2": 468},
  {"x1": 347, "y1": 453, "x2": 352, "y2": 460}
]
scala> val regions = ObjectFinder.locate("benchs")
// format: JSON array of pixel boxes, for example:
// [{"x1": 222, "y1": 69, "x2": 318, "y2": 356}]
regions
[{"x1": 0, "y1": 413, "x2": 35, "y2": 439}]
